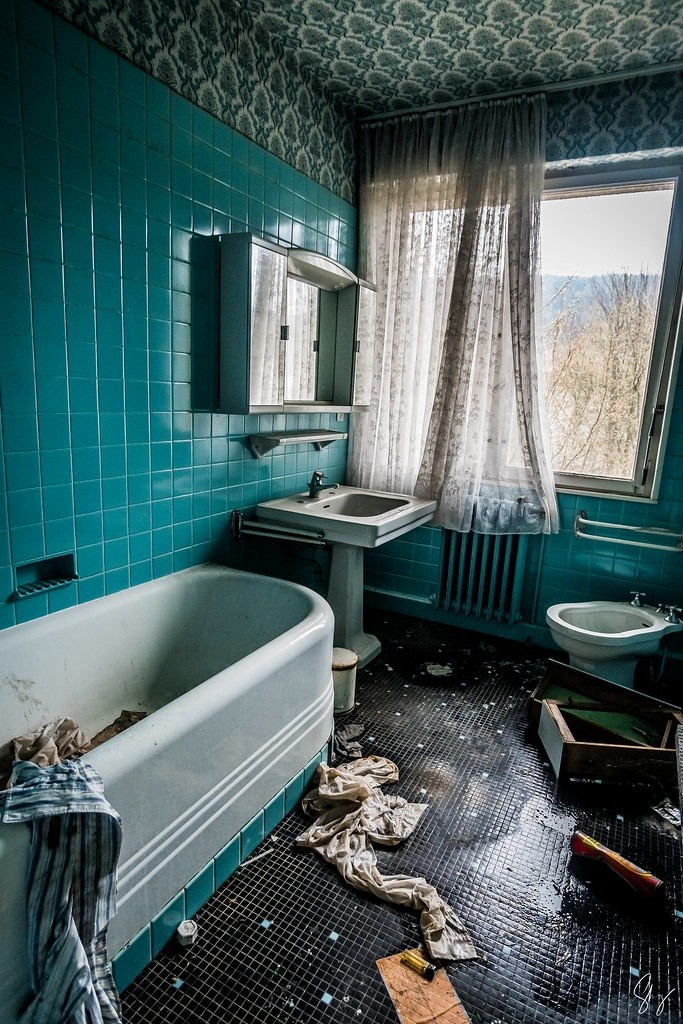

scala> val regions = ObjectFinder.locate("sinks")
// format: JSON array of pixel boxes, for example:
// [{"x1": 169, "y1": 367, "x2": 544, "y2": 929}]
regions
[
  {"x1": 255, "y1": 483, "x2": 437, "y2": 548},
  {"x1": 545, "y1": 601, "x2": 683, "y2": 695}
]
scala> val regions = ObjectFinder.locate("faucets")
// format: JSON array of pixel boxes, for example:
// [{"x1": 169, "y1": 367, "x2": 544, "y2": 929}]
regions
[
  {"x1": 307, "y1": 470, "x2": 339, "y2": 498},
  {"x1": 655, "y1": 601, "x2": 667, "y2": 613}
]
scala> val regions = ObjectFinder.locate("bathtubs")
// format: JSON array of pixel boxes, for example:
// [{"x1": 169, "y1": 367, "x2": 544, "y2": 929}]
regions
[{"x1": 1, "y1": 560, "x2": 336, "y2": 1024}]
